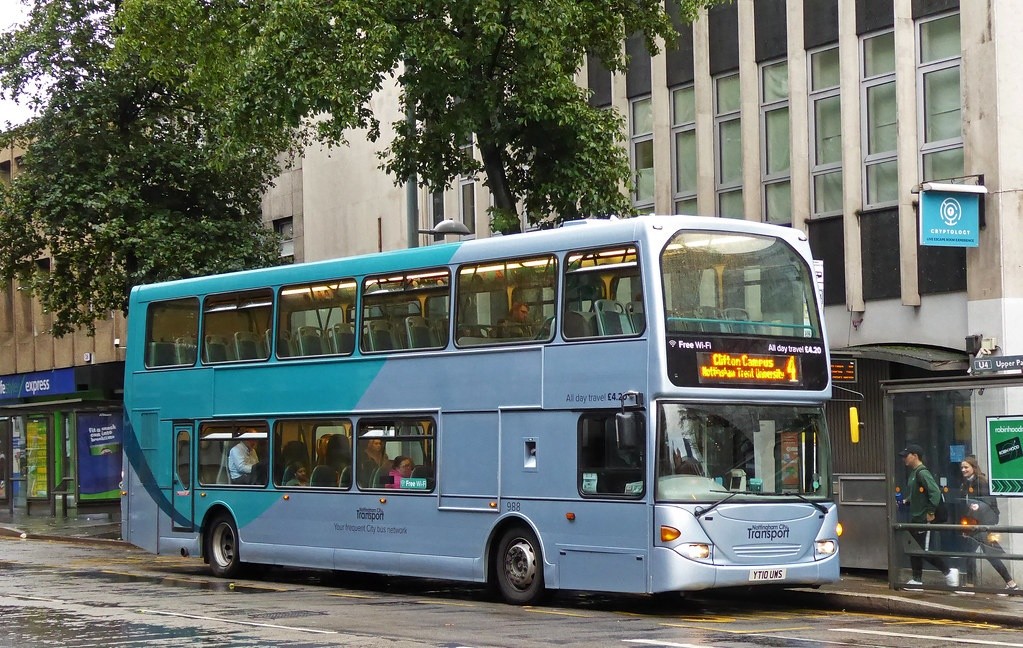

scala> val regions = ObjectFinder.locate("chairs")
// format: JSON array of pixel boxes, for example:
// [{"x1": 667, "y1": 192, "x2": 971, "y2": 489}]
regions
[
  {"x1": 147, "y1": 299, "x2": 762, "y2": 368},
  {"x1": 283, "y1": 440, "x2": 431, "y2": 491}
]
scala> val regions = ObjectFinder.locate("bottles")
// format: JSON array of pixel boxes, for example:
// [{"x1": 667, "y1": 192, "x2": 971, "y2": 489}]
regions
[{"x1": 895, "y1": 492, "x2": 905, "y2": 512}]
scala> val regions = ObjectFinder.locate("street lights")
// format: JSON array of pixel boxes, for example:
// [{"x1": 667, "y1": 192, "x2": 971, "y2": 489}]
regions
[{"x1": 405, "y1": 217, "x2": 470, "y2": 247}]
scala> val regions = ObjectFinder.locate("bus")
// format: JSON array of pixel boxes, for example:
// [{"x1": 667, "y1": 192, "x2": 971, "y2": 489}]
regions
[{"x1": 119, "y1": 214, "x2": 868, "y2": 606}]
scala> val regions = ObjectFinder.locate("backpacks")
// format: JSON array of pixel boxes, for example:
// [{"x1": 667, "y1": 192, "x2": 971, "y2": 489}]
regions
[{"x1": 916, "y1": 467, "x2": 948, "y2": 523}]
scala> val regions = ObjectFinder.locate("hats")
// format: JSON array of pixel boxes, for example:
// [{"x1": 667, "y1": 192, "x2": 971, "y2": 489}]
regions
[{"x1": 898, "y1": 445, "x2": 924, "y2": 457}]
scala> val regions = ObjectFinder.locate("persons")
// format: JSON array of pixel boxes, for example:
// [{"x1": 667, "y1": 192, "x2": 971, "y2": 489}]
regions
[
  {"x1": 501, "y1": 300, "x2": 537, "y2": 337},
  {"x1": 227, "y1": 428, "x2": 268, "y2": 484},
  {"x1": 284, "y1": 433, "x2": 413, "y2": 489},
  {"x1": 954, "y1": 457, "x2": 1019, "y2": 597},
  {"x1": 894, "y1": 444, "x2": 960, "y2": 592}
]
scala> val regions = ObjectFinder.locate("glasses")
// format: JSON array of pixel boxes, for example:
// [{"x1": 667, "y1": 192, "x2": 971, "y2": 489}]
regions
[
  {"x1": 519, "y1": 310, "x2": 528, "y2": 314},
  {"x1": 399, "y1": 464, "x2": 412, "y2": 469}
]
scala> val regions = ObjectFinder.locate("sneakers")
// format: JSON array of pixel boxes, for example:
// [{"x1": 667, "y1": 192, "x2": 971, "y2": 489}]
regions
[
  {"x1": 951, "y1": 585, "x2": 975, "y2": 596},
  {"x1": 944, "y1": 568, "x2": 959, "y2": 593},
  {"x1": 902, "y1": 580, "x2": 924, "y2": 591},
  {"x1": 996, "y1": 584, "x2": 1018, "y2": 596}
]
